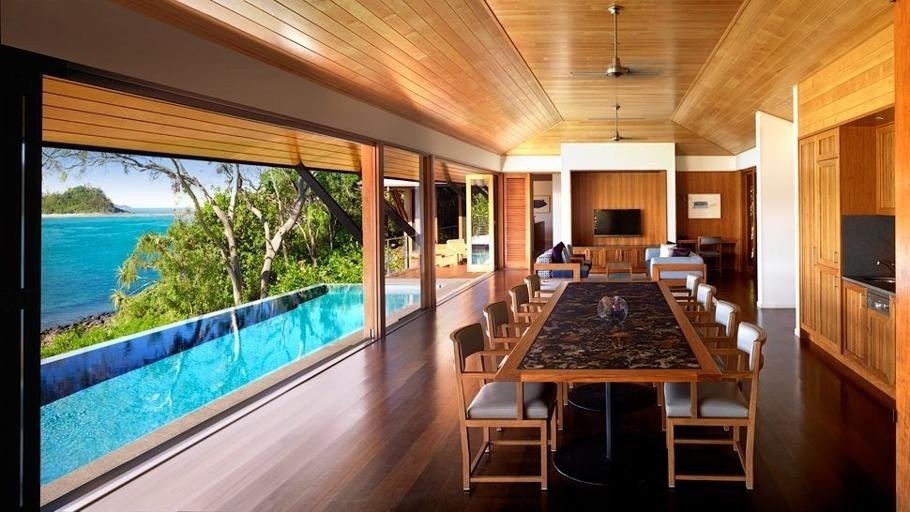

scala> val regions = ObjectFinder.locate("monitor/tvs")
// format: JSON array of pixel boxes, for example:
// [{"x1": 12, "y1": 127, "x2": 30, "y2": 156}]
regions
[{"x1": 593, "y1": 208, "x2": 641, "y2": 237}]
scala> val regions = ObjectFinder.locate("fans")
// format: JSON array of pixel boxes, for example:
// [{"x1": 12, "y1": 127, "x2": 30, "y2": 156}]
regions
[
  {"x1": 594, "y1": 4, "x2": 657, "y2": 79},
  {"x1": 602, "y1": 103, "x2": 646, "y2": 143}
]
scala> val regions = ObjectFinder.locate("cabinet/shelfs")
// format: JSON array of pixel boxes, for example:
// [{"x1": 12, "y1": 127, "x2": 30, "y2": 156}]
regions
[
  {"x1": 534, "y1": 220, "x2": 547, "y2": 257},
  {"x1": 818, "y1": 265, "x2": 843, "y2": 358},
  {"x1": 818, "y1": 125, "x2": 876, "y2": 272},
  {"x1": 800, "y1": 133, "x2": 818, "y2": 336},
  {"x1": 875, "y1": 120, "x2": 897, "y2": 217},
  {"x1": 841, "y1": 279, "x2": 895, "y2": 389}
]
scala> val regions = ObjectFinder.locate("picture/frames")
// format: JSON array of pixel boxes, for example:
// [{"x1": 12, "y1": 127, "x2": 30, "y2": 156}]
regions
[{"x1": 533, "y1": 194, "x2": 551, "y2": 214}]
